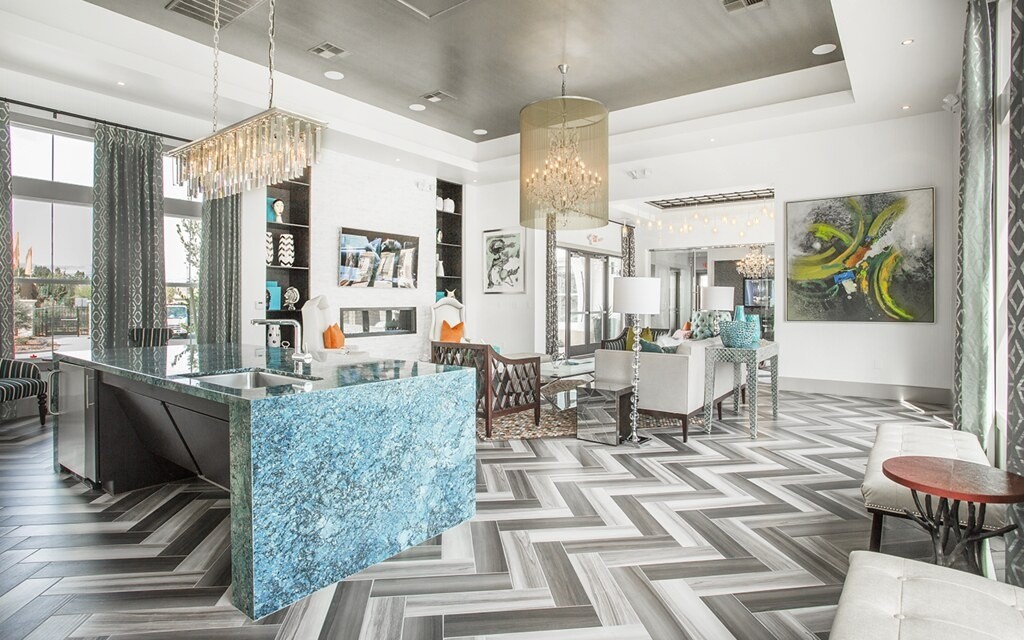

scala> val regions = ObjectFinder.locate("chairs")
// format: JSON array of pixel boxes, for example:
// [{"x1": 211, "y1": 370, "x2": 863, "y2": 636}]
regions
[
  {"x1": 429, "y1": 298, "x2": 486, "y2": 344},
  {"x1": 428, "y1": 341, "x2": 541, "y2": 437},
  {"x1": 0, "y1": 357, "x2": 47, "y2": 425},
  {"x1": 300, "y1": 294, "x2": 365, "y2": 360}
]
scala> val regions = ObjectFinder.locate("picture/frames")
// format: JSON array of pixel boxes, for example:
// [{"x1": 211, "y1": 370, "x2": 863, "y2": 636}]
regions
[
  {"x1": 785, "y1": 186, "x2": 935, "y2": 324},
  {"x1": 483, "y1": 226, "x2": 525, "y2": 292}
]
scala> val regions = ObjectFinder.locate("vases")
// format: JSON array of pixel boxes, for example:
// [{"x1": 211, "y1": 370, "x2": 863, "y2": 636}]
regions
[
  {"x1": 266, "y1": 232, "x2": 274, "y2": 265},
  {"x1": 436, "y1": 178, "x2": 463, "y2": 306},
  {"x1": 717, "y1": 305, "x2": 761, "y2": 348},
  {"x1": 278, "y1": 234, "x2": 295, "y2": 267},
  {"x1": 267, "y1": 323, "x2": 281, "y2": 347}
]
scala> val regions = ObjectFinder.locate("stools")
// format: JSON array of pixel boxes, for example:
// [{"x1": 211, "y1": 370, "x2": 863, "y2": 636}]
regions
[{"x1": 577, "y1": 381, "x2": 632, "y2": 446}]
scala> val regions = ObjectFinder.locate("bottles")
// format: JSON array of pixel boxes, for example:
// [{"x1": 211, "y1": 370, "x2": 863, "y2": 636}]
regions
[
  {"x1": 732, "y1": 305, "x2": 746, "y2": 322},
  {"x1": 437, "y1": 194, "x2": 455, "y2": 213},
  {"x1": 265, "y1": 231, "x2": 273, "y2": 265},
  {"x1": 277, "y1": 233, "x2": 295, "y2": 267},
  {"x1": 436, "y1": 261, "x2": 444, "y2": 276},
  {"x1": 267, "y1": 325, "x2": 281, "y2": 347}
]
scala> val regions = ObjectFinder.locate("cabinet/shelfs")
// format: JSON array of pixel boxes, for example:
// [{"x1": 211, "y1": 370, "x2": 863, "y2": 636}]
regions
[{"x1": 261, "y1": 164, "x2": 312, "y2": 346}]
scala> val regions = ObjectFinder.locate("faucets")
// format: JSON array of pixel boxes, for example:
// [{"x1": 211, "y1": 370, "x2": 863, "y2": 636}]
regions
[{"x1": 249, "y1": 318, "x2": 313, "y2": 375}]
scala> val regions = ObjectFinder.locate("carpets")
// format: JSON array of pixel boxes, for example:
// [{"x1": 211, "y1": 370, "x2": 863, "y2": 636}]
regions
[{"x1": 476, "y1": 395, "x2": 705, "y2": 444}]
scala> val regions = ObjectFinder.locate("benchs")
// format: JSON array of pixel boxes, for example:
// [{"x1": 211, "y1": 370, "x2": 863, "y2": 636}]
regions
[
  {"x1": 595, "y1": 310, "x2": 746, "y2": 444},
  {"x1": 829, "y1": 423, "x2": 1024, "y2": 640}
]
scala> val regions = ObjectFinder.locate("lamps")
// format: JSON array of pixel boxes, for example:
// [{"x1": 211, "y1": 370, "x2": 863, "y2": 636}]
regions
[
  {"x1": 622, "y1": 188, "x2": 775, "y2": 277},
  {"x1": 700, "y1": 287, "x2": 735, "y2": 312},
  {"x1": 613, "y1": 277, "x2": 661, "y2": 442},
  {"x1": 167, "y1": 1, "x2": 328, "y2": 205},
  {"x1": 518, "y1": 64, "x2": 609, "y2": 228}
]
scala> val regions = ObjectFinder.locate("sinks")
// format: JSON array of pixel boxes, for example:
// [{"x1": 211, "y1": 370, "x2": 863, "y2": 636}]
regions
[{"x1": 186, "y1": 371, "x2": 313, "y2": 390}]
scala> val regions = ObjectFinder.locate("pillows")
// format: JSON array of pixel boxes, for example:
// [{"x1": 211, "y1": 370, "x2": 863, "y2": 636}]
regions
[
  {"x1": 439, "y1": 320, "x2": 465, "y2": 343},
  {"x1": 324, "y1": 322, "x2": 344, "y2": 350}
]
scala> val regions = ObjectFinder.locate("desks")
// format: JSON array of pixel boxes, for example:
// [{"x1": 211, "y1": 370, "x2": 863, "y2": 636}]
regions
[
  {"x1": 539, "y1": 358, "x2": 595, "y2": 413},
  {"x1": 880, "y1": 455, "x2": 1023, "y2": 581},
  {"x1": 703, "y1": 339, "x2": 777, "y2": 438}
]
